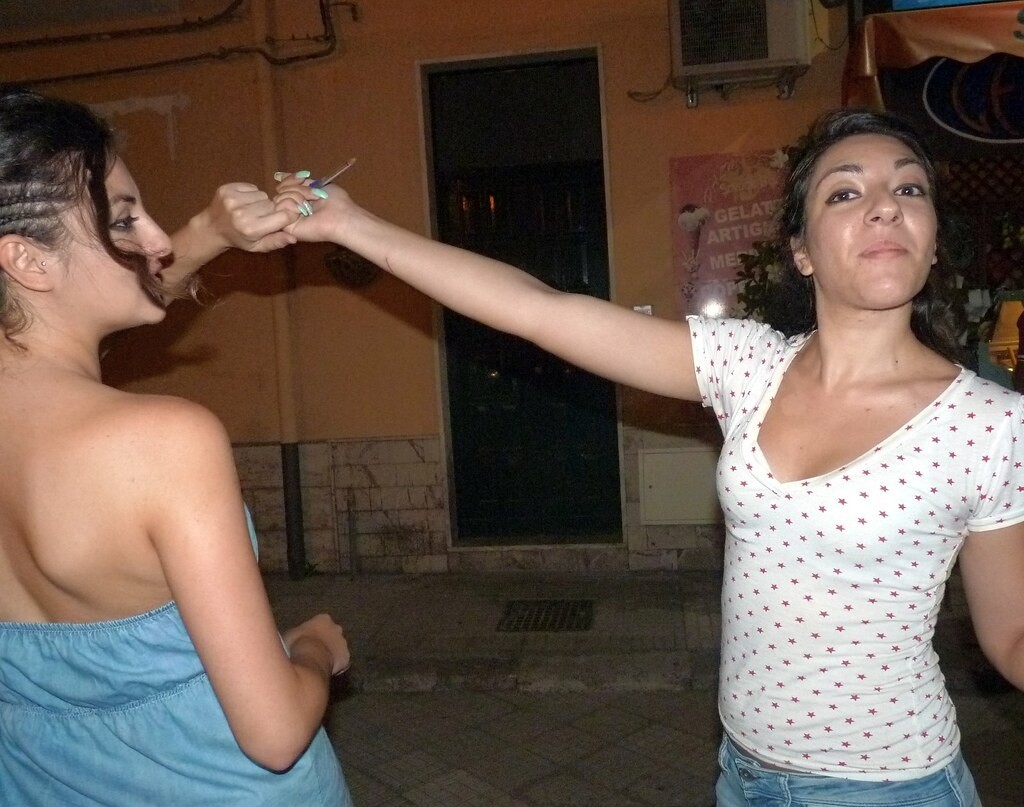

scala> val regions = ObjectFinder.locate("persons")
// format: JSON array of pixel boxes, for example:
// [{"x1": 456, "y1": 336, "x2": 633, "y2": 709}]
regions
[
  {"x1": 0, "y1": 94, "x2": 354, "y2": 807},
  {"x1": 272, "y1": 106, "x2": 1024, "y2": 806}
]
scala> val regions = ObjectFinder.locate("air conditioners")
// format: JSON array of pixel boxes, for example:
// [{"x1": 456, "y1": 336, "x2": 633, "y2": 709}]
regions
[{"x1": 667, "y1": 0, "x2": 811, "y2": 89}]
